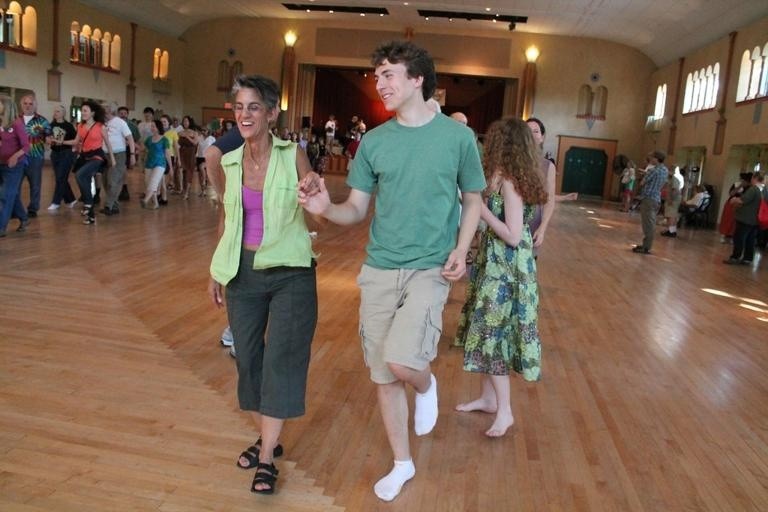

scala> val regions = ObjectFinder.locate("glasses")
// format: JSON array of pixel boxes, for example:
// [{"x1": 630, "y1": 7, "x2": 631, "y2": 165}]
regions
[{"x1": 231, "y1": 103, "x2": 268, "y2": 115}]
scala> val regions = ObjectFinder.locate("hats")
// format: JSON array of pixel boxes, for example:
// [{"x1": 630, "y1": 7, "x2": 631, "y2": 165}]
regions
[{"x1": 647, "y1": 150, "x2": 665, "y2": 161}]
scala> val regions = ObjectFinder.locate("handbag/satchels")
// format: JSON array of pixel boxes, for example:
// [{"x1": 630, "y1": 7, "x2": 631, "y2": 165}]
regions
[
  {"x1": 758, "y1": 198, "x2": 768, "y2": 230},
  {"x1": 72, "y1": 151, "x2": 85, "y2": 172}
]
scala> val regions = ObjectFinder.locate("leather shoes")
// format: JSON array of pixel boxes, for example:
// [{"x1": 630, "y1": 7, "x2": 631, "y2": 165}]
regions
[
  {"x1": 113, "y1": 207, "x2": 120, "y2": 214},
  {"x1": 739, "y1": 259, "x2": 750, "y2": 265},
  {"x1": 99, "y1": 206, "x2": 113, "y2": 216},
  {"x1": 723, "y1": 257, "x2": 739, "y2": 264}
]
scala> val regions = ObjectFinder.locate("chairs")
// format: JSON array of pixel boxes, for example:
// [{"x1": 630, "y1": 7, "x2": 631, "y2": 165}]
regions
[{"x1": 688, "y1": 195, "x2": 711, "y2": 229}]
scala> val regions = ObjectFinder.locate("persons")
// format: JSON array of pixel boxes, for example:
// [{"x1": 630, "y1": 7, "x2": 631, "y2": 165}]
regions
[
  {"x1": 721, "y1": 171, "x2": 768, "y2": 265},
  {"x1": 295, "y1": 40, "x2": 489, "y2": 504},
  {"x1": 451, "y1": 112, "x2": 468, "y2": 125},
  {"x1": 271, "y1": 113, "x2": 367, "y2": 177},
  {"x1": 618, "y1": 150, "x2": 713, "y2": 254},
  {"x1": 2, "y1": 94, "x2": 237, "y2": 237},
  {"x1": 453, "y1": 116, "x2": 548, "y2": 439},
  {"x1": 210, "y1": 74, "x2": 328, "y2": 497}
]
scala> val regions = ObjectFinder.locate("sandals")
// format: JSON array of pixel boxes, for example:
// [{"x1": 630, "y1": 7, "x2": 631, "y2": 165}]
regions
[
  {"x1": 80, "y1": 200, "x2": 95, "y2": 216},
  {"x1": 83, "y1": 216, "x2": 95, "y2": 225},
  {"x1": 237, "y1": 438, "x2": 283, "y2": 469},
  {"x1": 251, "y1": 462, "x2": 279, "y2": 494}
]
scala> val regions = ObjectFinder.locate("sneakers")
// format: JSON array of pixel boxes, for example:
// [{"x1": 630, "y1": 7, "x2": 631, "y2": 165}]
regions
[
  {"x1": 157, "y1": 194, "x2": 161, "y2": 199},
  {"x1": 221, "y1": 325, "x2": 234, "y2": 346},
  {"x1": 229, "y1": 345, "x2": 235, "y2": 357},
  {"x1": 633, "y1": 246, "x2": 648, "y2": 254},
  {"x1": 636, "y1": 245, "x2": 642, "y2": 248},
  {"x1": 47, "y1": 204, "x2": 60, "y2": 210},
  {"x1": 26, "y1": 212, "x2": 37, "y2": 218},
  {"x1": 68, "y1": 199, "x2": 78, "y2": 208},
  {"x1": 661, "y1": 230, "x2": 676, "y2": 238},
  {"x1": 158, "y1": 198, "x2": 169, "y2": 206},
  {"x1": 16, "y1": 219, "x2": 31, "y2": 232}
]
